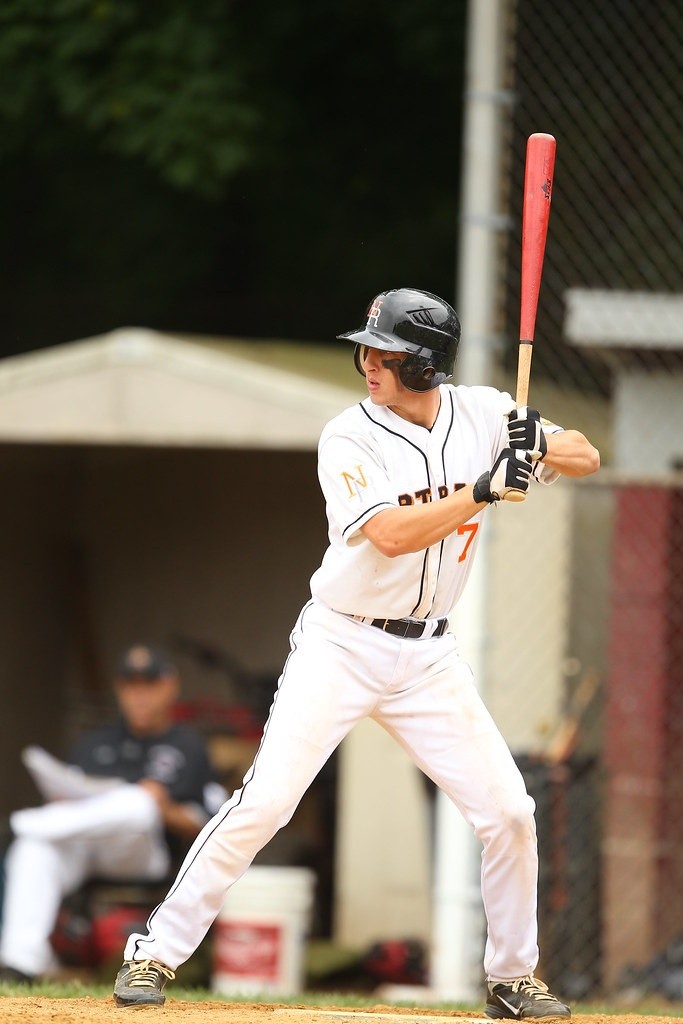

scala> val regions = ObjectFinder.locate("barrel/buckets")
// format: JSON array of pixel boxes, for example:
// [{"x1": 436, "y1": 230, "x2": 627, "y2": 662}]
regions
[{"x1": 212, "y1": 865, "x2": 317, "y2": 1003}]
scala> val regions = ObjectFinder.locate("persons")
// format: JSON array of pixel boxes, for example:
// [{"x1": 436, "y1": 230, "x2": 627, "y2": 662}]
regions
[
  {"x1": 0, "y1": 644, "x2": 234, "y2": 988},
  {"x1": 111, "y1": 289, "x2": 599, "y2": 1021}
]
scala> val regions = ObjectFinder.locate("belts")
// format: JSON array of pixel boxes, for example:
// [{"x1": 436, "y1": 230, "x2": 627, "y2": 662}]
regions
[{"x1": 350, "y1": 614, "x2": 447, "y2": 639}]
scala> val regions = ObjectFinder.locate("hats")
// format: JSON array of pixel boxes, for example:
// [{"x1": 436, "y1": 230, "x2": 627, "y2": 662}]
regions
[{"x1": 114, "y1": 643, "x2": 176, "y2": 682}]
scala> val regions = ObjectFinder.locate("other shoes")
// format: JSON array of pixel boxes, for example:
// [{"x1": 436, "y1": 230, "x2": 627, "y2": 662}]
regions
[{"x1": 0, "y1": 968, "x2": 31, "y2": 987}]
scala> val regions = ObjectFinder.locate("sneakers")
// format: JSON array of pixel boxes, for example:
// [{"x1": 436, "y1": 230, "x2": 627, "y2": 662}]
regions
[
  {"x1": 113, "y1": 960, "x2": 175, "y2": 1008},
  {"x1": 484, "y1": 975, "x2": 571, "y2": 1020}
]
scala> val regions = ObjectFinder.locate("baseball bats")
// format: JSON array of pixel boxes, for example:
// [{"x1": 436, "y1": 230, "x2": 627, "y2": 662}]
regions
[{"x1": 495, "y1": 132, "x2": 564, "y2": 505}]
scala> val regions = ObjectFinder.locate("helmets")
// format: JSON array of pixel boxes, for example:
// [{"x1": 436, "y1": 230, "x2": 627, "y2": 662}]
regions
[{"x1": 337, "y1": 288, "x2": 461, "y2": 393}]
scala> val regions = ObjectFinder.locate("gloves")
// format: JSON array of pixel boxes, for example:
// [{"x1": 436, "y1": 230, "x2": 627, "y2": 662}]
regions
[
  {"x1": 473, "y1": 448, "x2": 542, "y2": 505},
  {"x1": 503, "y1": 406, "x2": 547, "y2": 462}
]
